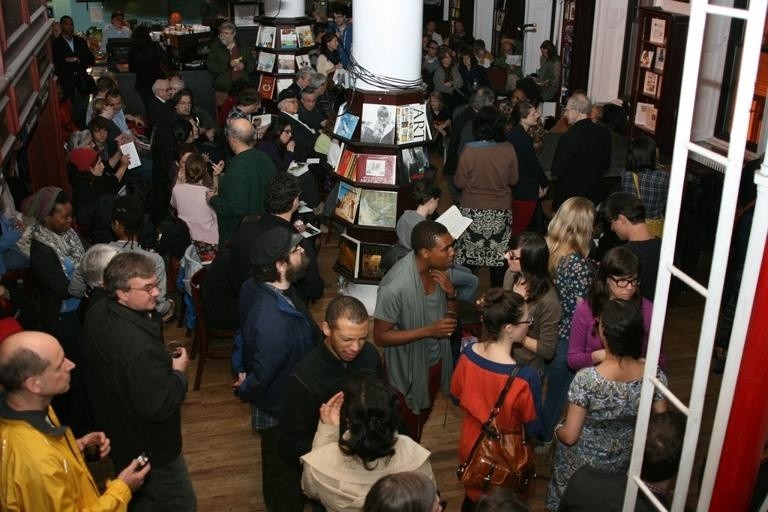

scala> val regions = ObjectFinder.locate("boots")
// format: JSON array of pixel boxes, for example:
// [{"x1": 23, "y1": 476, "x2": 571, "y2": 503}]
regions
[{"x1": 534, "y1": 439, "x2": 555, "y2": 480}]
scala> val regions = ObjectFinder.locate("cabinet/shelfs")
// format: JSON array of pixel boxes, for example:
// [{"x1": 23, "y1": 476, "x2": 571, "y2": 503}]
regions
[{"x1": 625, "y1": 6, "x2": 690, "y2": 166}]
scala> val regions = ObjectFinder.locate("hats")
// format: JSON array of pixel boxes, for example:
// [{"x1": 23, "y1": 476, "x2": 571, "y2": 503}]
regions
[
  {"x1": 278, "y1": 88, "x2": 296, "y2": 101},
  {"x1": 111, "y1": 10, "x2": 124, "y2": 17},
  {"x1": 69, "y1": 148, "x2": 98, "y2": 172},
  {"x1": 248, "y1": 227, "x2": 304, "y2": 272},
  {"x1": 264, "y1": 170, "x2": 303, "y2": 205},
  {"x1": 20, "y1": 185, "x2": 63, "y2": 222}
]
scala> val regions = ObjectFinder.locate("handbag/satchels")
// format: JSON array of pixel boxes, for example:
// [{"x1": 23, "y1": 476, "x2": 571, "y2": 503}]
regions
[
  {"x1": 456, "y1": 422, "x2": 536, "y2": 495},
  {"x1": 644, "y1": 217, "x2": 664, "y2": 238}
]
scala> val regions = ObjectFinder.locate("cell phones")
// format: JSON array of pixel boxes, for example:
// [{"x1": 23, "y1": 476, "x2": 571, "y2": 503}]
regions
[{"x1": 137, "y1": 451, "x2": 151, "y2": 471}]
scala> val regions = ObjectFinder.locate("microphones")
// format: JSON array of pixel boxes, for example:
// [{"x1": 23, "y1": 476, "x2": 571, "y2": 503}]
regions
[{"x1": 523, "y1": 24, "x2": 536, "y2": 27}]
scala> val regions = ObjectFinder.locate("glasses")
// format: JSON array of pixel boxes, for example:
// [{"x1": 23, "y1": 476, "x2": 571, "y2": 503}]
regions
[
  {"x1": 129, "y1": 276, "x2": 161, "y2": 292},
  {"x1": 158, "y1": 87, "x2": 171, "y2": 91},
  {"x1": 503, "y1": 314, "x2": 532, "y2": 329},
  {"x1": 282, "y1": 129, "x2": 293, "y2": 134},
  {"x1": 607, "y1": 275, "x2": 641, "y2": 287}
]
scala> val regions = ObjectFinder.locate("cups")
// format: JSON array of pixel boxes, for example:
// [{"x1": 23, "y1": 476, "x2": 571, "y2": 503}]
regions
[
  {"x1": 166, "y1": 340, "x2": 183, "y2": 358},
  {"x1": 443, "y1": 310, "x2": 459, "y2": 320}
]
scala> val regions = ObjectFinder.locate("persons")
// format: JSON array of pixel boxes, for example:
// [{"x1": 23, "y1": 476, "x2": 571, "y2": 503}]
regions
[
  {"x1": 1, "y1": 10, "x2": 445, "y2": 512},
  {"x1": 371, "y1": 14, "x2": 689, "y2": 512}
]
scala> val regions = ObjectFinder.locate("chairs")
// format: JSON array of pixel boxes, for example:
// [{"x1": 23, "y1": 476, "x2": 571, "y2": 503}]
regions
[{"x1": 186, "y1": 263, "x2": 241, "y2": 393}]
